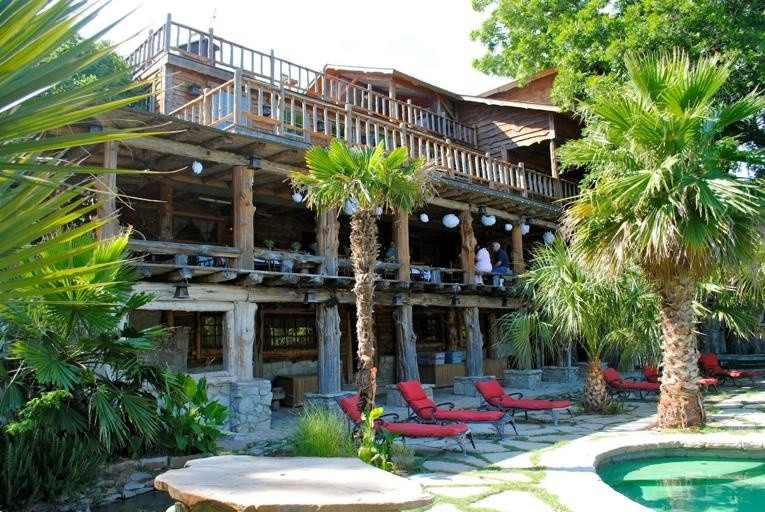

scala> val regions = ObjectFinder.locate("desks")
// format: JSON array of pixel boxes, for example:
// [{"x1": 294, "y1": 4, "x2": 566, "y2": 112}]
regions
[{"x1": 278, "y1": 375, "x2": 318, "y2": 408}]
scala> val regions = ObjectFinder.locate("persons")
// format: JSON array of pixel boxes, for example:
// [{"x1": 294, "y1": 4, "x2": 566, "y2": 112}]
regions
[
  {"x1": 489, "y1": 242, "x2": 509, "y2": 286},
  {"x1": 387, "y1": 240, "x2": 396, "y2": 261},
  {"x1": 473, "y1": 241, "x2": 492, "y2": 284}
]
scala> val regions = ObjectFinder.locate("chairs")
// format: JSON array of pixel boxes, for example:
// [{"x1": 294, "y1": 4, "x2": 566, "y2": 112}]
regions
[
  {"x1": 400, "y1": 380, "x2": 519, "y2": 442},
  {"x1": 383, "y1": 258, "x2": 397, "y2": 278},
  {"x1": 475, "y1": 376, "x2": 579, "y2": 427},
  {"x1": 336, "y1": 394, "x2": 476, "y2": 457},
  {"x1": 601, "y1": 353, "x2": 763, "y2": 404}
]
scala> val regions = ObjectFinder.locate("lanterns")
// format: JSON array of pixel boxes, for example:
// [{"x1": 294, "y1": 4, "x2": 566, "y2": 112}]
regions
[
  {"x1": 292, "y1": 194, "x2": 303, "y2": 204},
  {"x1": 521, "y1": 224, "x2": 530, "y2": 235},
  {"x1": 504, "y1": 223, "x2": 512, "y2": 232},
  {"x1": 481, "y1": 215, "x2": 497, "y2": 226},
  {"x1": 542, "y1": 231, "x2": 555, "y2": 244},
  {"x1": 420, "y1": 213, "x2": 429, "y2": 222},
  {"x1": 441, "y1": 214, "x2": 460, "y2": 229},
  {"x1": 374, "y1": 206, "x2": 382, "y2": 215},
  {"x1": 343, "y1": 199, "x2": 362, "y2": 217},
  {"x1": 192, "y1": 162, "x2": 204, "y2": 175}
]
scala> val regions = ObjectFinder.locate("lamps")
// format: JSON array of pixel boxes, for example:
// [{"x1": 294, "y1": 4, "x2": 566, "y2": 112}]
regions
[
  {"x1": 189, "y1": 82, "x2": 327, "y2": 131},
  {"x1": 247, "y1": 154, "x2": 264, "y2": 169},
  {"x1": 303, "y1": 288, "x2": 511, "y2": 307},
  {"x1": 172, "y1": 281, "x2": 192, "y2": 298},
  {"x1": 477, "y1": 203, "x2": 532, "y2": 225}
]
